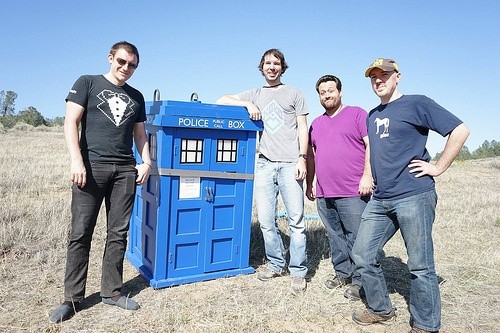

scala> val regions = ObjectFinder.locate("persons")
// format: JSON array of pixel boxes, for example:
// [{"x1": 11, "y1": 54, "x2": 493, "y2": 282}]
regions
[
  {"x1": 219, "y1": 49, "x2": 310, "y2": 293},
  {"x1": 306, "y1": 76, "x2": 373, "y2": 300},
  {"x1": 346, "y1": 57, "x2": 469, "y2": 333},
  {"x1": 49, "y1": 41, "x2": 153, "y2": 323}
]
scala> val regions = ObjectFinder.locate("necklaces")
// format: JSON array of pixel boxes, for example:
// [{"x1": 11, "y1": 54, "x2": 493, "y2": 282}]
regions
[{"x1": 263, "y1": 83, "x2": 283, "y2": 88}]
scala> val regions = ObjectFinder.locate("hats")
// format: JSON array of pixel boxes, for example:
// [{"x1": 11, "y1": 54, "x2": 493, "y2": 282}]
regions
[{"x1": 364, "y1": 58, "x2": 400, "y2": 78}]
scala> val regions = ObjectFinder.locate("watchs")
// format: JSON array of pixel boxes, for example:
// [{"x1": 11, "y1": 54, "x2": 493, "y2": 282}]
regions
[{"x1": 299, "y1": 154, "x2": 308, "y2": 161}]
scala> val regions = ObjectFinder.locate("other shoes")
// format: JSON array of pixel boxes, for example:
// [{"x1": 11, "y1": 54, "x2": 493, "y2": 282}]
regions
[
  {"x1": 324, "y1": 275, "x2": 350, "y2": 289},
  {"x1": 50, "y1": 300, "x2": 80, "y2": 322},
  {"x1": 102, "y1": 294, "x2": 141, "y2": 310},
  {"x1": 344, "y1": 284, "x2": 366, "y2": 301}
]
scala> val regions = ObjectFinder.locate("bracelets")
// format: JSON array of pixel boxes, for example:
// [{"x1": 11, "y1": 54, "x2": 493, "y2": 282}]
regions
[{"x1": 144, "y1": 161, "x2": 152, "y2": 169}]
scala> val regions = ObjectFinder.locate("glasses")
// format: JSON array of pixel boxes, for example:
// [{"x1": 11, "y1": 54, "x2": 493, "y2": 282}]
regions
[{"x1": 112, "y1": 54, "x2": 137, "y2": 69}]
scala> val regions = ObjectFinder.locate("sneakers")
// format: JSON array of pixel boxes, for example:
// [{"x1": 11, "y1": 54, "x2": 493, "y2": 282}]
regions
[
  {"x1": 353, "y1": 308, "x2": 396, "y2": 326},
  {"x1": 409, "y1": 327, "x2": 439, "y2": 333},
  {"x1": 291, "y1": 276, "x2": 306, "y2": 292},
  {"x1": 258, "y1": 268, "x2": 291, "y2": 281}
]
